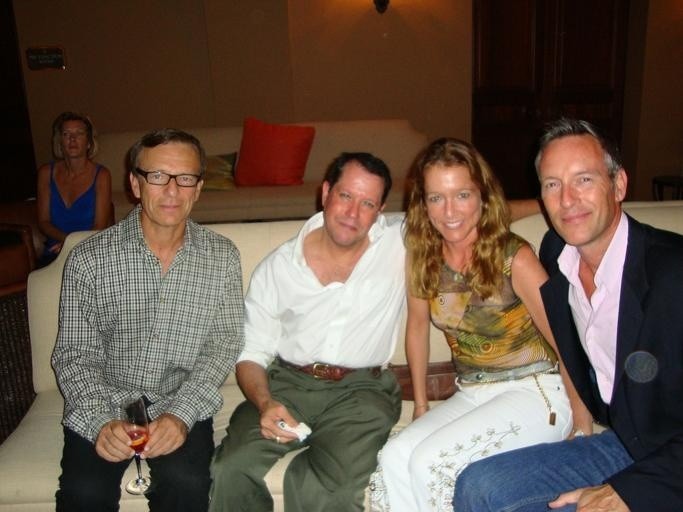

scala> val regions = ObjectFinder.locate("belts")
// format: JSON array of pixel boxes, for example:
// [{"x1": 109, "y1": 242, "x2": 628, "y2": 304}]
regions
[{"x1": 279, "y1": 356, "x2": 382, "y2": 382}]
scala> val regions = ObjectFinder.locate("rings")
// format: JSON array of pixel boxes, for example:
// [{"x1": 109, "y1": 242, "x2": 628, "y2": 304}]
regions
[{"x1": 276, "y1": 436, "x2": 279, "y2": 443}]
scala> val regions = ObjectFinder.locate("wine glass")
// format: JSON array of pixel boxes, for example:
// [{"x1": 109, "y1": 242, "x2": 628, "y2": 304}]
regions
[{"x1": 113, "y1": 396, "x2": 158, "y2": 496}]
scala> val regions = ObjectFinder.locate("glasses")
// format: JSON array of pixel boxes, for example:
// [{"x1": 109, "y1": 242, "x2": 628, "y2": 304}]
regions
[{"x1": 133, "y1": 165, "x2": 203, "y2": 188}]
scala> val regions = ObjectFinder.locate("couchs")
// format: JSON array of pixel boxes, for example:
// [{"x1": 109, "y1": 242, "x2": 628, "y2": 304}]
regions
[{"x1": 1, "y1": 201, "x2": 682, "y2": 510}]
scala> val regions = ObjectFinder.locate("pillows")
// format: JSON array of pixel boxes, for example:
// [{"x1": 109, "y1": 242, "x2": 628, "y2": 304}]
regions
[{"x1": 232, "y1": 116, "x2": 316, "y2": 187}]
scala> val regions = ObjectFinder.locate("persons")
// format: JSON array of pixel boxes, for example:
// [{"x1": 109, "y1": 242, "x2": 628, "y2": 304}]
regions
[
  {"x1": 361, "y1": 137, "x2": 595, "y2": 512},
  {"x1": 451, "y1": 115, "x2": 683, "y2": 512},
  {"x1": 204, "y1": 151, "x2": 408, "y2": 512},
  {"x1": 32, "y1": 111, "x2": 113, "y2": 270},
  {"x1": 48, "y1": 128, "x2": 248, "y2": 511}
]
